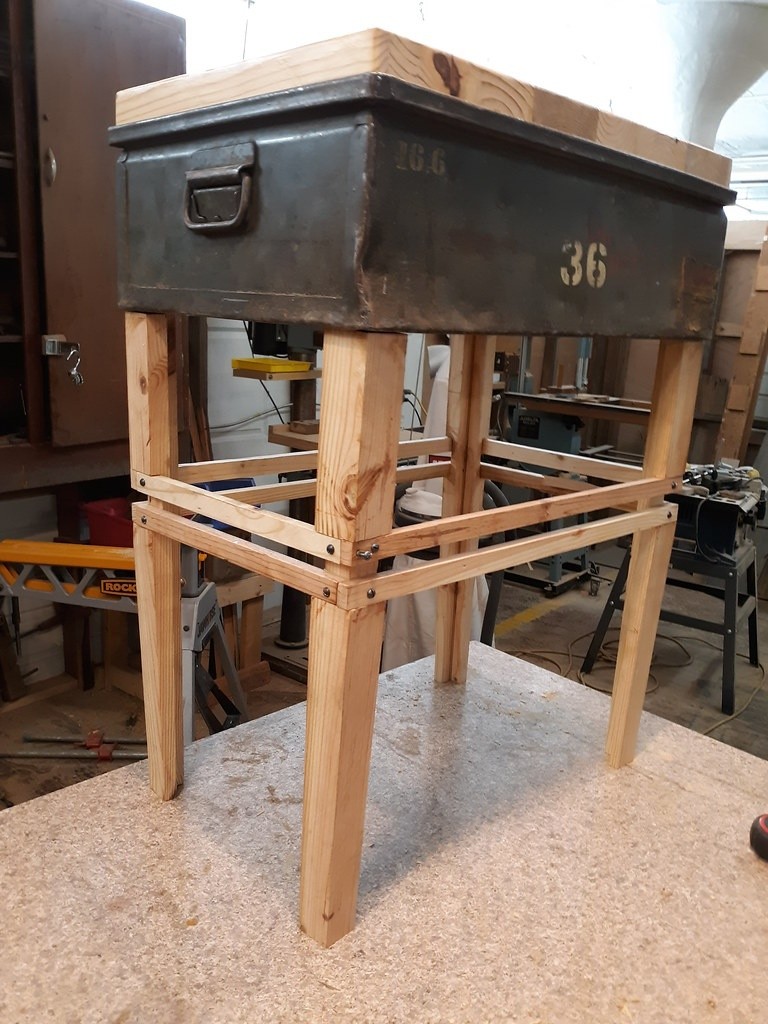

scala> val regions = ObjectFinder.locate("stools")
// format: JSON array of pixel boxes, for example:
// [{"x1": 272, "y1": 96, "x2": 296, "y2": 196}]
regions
[{"x1": 107, "y1": 25, "x2": 738, "y2": 945}]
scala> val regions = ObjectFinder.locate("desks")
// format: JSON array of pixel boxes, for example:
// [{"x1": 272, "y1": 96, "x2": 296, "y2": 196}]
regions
[{"x1": 0, "y1": 640, "x2": 768, "y2": 1023}]
